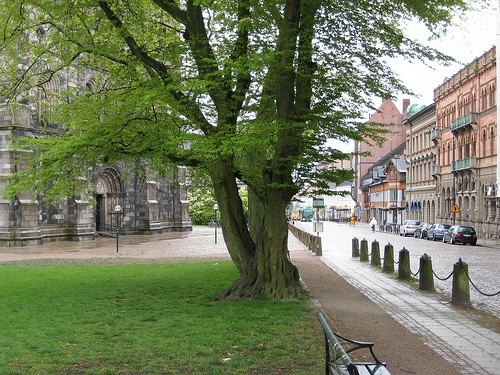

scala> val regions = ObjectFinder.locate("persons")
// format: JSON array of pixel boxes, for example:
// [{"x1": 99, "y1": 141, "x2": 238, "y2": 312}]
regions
[{"x1": 370, "y1": 216, "x2": 377, "y2": 230}]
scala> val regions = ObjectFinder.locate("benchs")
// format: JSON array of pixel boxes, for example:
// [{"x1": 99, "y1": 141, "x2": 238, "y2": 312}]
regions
[{"x1": 317, "y1": 311, "x2": 390, "y2": 375}]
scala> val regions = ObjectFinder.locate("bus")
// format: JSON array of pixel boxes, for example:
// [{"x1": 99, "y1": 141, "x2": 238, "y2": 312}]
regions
[{"x1": 299, "y1": 207, "x2": 315, "y2": 222}]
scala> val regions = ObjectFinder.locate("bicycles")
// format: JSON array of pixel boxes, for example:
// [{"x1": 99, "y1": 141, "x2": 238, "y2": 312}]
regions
[{"x1": 371, "y1": 224, "x2": 375, "y2": 232}]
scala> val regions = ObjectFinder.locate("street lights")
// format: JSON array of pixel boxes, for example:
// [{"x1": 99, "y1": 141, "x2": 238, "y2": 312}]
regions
[
  {"x1": 115, "y1": 205, "x2": 121, "y2": 253},
  {"x1": 213, "y1": 204, "x2": 219, "y2": 244}
]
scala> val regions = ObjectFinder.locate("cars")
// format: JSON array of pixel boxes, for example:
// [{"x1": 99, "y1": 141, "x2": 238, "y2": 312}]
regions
[
  {"x1": 442, "y1": 225, "x2": 477, "y2": 245},
  {"x1": 413, "y1": 222, "x2": 433, "y2": 238},
  {"x1": 399, "y1": 220, "x2": 422, "y2": 237},
  {"x1": 427, "y1": 223, "x2": 451, "y2": 241}
]
